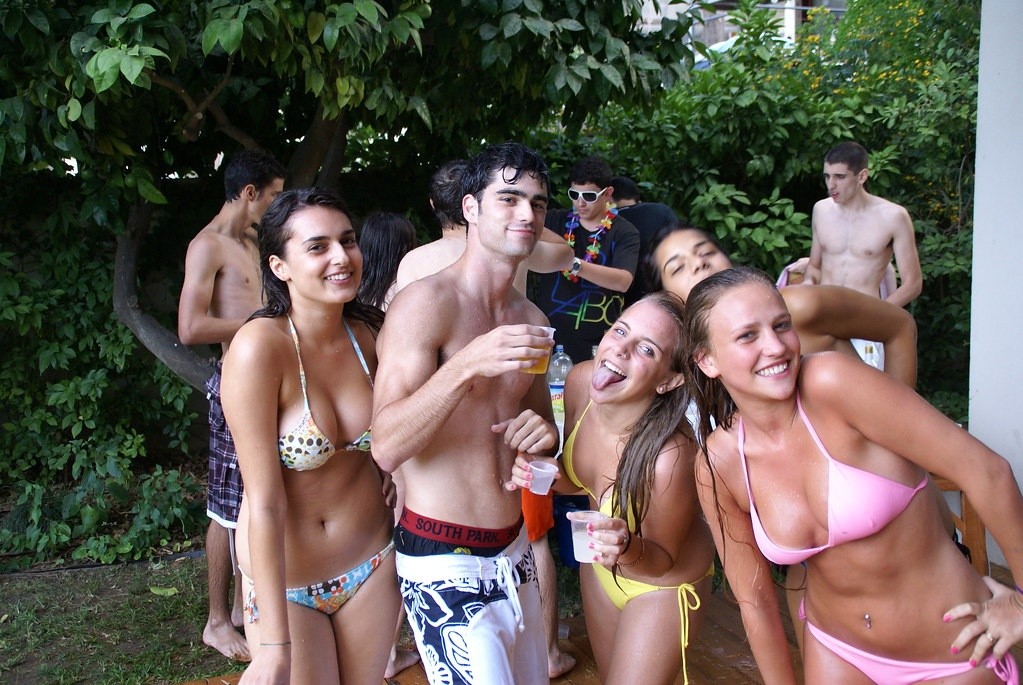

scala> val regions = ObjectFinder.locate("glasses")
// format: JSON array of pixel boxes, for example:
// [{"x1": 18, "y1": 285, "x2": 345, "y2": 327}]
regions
[{"x1": 568, "y1": 186, "x2": 608, "y2": 204}]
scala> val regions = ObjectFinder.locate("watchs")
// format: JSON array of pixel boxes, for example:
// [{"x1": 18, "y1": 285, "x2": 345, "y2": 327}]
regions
[{"x1": 568, "y1": 256, "x2": 582, "y2": 276}]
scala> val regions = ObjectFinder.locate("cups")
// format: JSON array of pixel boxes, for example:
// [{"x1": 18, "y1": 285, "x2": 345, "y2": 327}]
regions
[
  {"x1": 518, "y1": 325, "x2": 557, "y2": 375},
  {"x1": 529, "y1": 461, "x2": 559, "y2": 496},
  {"x1": 567, "y1": 509, "x2": 611, "y2": 565}
]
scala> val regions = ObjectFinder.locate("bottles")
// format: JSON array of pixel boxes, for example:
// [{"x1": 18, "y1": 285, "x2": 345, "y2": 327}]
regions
[{"x1": 547, "y1": 345, "x2": 573, "y2": 424}]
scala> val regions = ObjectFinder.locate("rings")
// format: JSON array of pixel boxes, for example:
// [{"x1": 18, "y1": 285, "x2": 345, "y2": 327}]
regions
[
  {"x1": 622, "y1": 533, "x2": 627, "y2": 543},
  {"x1": 985, "y1": 632, "x2": 996, "y2": 644}
]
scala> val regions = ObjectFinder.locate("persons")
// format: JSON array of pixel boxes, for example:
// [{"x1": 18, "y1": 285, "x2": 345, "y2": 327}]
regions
[
  {"x1": 644, "y1": 214, "x2": 919, "y2": 448},
  {"x1": 381, "y1": 157, "x2": 574, "y2": 313},
  {"x1": 538, "y1": 152, "x2": 639, "y2": 367},
  {"x1": 220, "y1": 185, "x2": 397, "y2": 685},
  {"x1": 177, "y1": 146, "x2": 287, "y2": 664},
  {"x1": 788, "y1": 141, "x2": 922, "y2": 372},
  {"x1": 369, "y1": 141, "x2": 557, "y2": 685},
  {"x1": 680, "y1": 264, "x2": 1023, "y2": 685},
  {"x1": 356, "y1": 210, "x2": 417, "y2": 310},
  {"x1": 609, "y1": 176, "x2": 680, "y2": 312}
]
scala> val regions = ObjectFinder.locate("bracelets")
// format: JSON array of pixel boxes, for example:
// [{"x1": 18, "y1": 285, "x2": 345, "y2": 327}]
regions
[
  {"x1": 550, "y1": 479, "x2": 556, "y2": 487},
  {"x1": 617, "y1": 536, "x2": 645, "y2": 567},
  {"x1": 621, "y1": 530, "x2": 631, "y2": 555},
  {"x1": 1014, "y1": 584, "x2": 1023, "y2": 595}
]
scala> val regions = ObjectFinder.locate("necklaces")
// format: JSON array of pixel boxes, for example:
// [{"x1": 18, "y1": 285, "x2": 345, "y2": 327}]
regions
[{"x1": 564, "y1": 204, "x2": 617, "y2": 281}]
